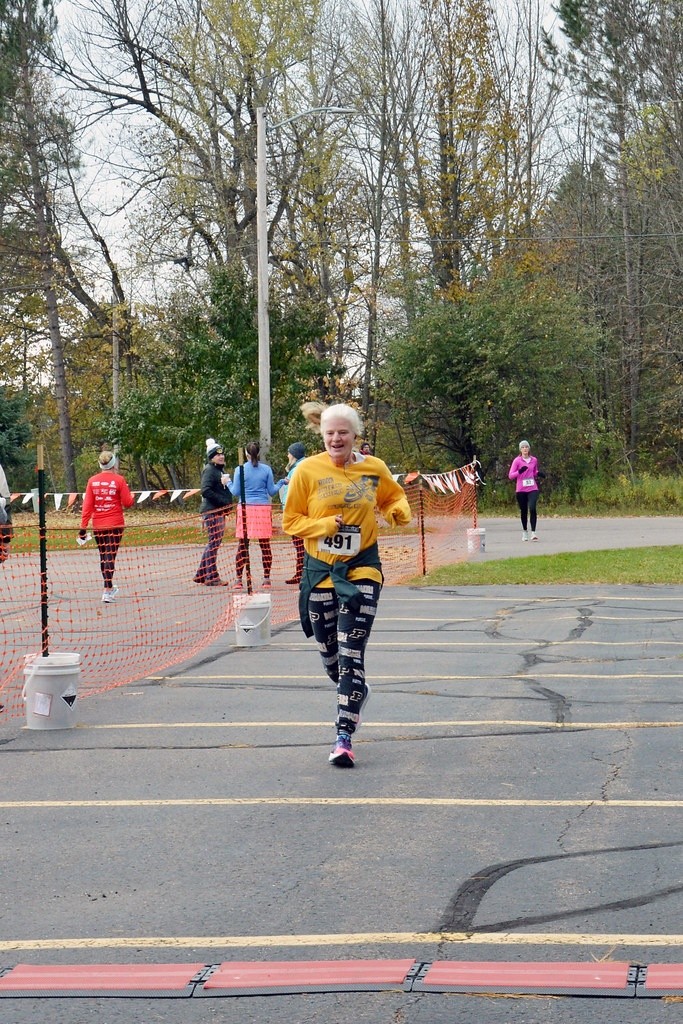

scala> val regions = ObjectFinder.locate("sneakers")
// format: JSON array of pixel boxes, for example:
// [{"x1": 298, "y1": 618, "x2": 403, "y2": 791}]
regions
[
  {"x1": 329, "y1": 734, "x2": 355, "y2": 768},
  {"x1": 355, "y1": 683, "x2": 372, "y2": 731},
  {"x1": 101, "y1": 585, "x2": 117, "y2": 596},
  {"x1": 102, "y1": 594, "x2": 116, "y2": 602}
]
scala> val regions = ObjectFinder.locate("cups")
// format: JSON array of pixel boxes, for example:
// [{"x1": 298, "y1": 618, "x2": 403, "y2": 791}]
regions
[{"x1": 222, "y1": 474, "x2": 230, "y2": 478}]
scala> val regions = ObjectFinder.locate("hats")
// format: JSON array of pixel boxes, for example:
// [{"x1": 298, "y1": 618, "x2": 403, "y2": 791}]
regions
[
  {"x1": 288, "y1": 442, "x2": 305, "y2": 460},
  {"x1": 205, "y1": 439, "x2": 223, "y2": 461}
]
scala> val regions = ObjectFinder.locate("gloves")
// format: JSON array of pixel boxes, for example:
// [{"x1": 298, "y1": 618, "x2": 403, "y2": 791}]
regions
[
  {"x1": 537, "y1": 471, "x2": 546, "y2": 478},
  {"x1": 79, "y1": 530, "x2": 87, "y2": 540},
  {"x1": 518, "y1": 466, "x2": 528, "y2": 474}
]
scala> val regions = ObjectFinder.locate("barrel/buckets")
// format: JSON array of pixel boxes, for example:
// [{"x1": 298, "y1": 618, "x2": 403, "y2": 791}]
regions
[
  {"x1": 21, "y1": 652, "x2": 82, "y2": 729},
  {"x1": 466, "y1": 527, "x2": 486, "y2": 553},
  {"x1": 233, "y1": 594, "x2": 272, "y2": 648}
]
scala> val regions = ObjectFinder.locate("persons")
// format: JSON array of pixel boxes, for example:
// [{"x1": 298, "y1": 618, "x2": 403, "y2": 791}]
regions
[
  {"x1": 193, "y1": 438, "x2": 232, "y2": 585},
  {"x1": 0, "y1": 464, "x2": 13, "y2": 565},
  {"x1": 221, "y1": 442, "x2": 288, "y2": 589},
  {"x1": 282, "y1": 404, "x2": 411, "y2": 765},
  {"x1": 79, "y1": 450, "x2": 135, "y2": 602},
  {"x1": 508, "y1": 441, "x2": 546, "y2": 542},
  {"x1": 359, "y1": 442, "x2": 373, "y2": 455},
  {"x1": 281, "y1": 442, "x2": 306, "y2": 584}
]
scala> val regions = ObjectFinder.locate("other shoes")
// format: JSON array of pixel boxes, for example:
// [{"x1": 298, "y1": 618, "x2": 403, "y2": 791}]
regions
[
  {"x1": 285, "y1": 575, "x2": 302, "y2": 583},
  {"x1": 206, "y1": 578, "x2": 228, "y2": 585},
  {"x1": 229, "y1": 579, "x2": 242, "y2": 588},
  {"x1": 193, "y1": 574, "x2": 207, "y2": 582},
  {"x1": 261, "y1": 579, "x2": 272, "y2": 586},
  {"x1": 523, "y1": 531, "x2": 529, "y2": 541},
  {"x1": 531, "y1": 532, "x2": 538, "y2": 540}
]
